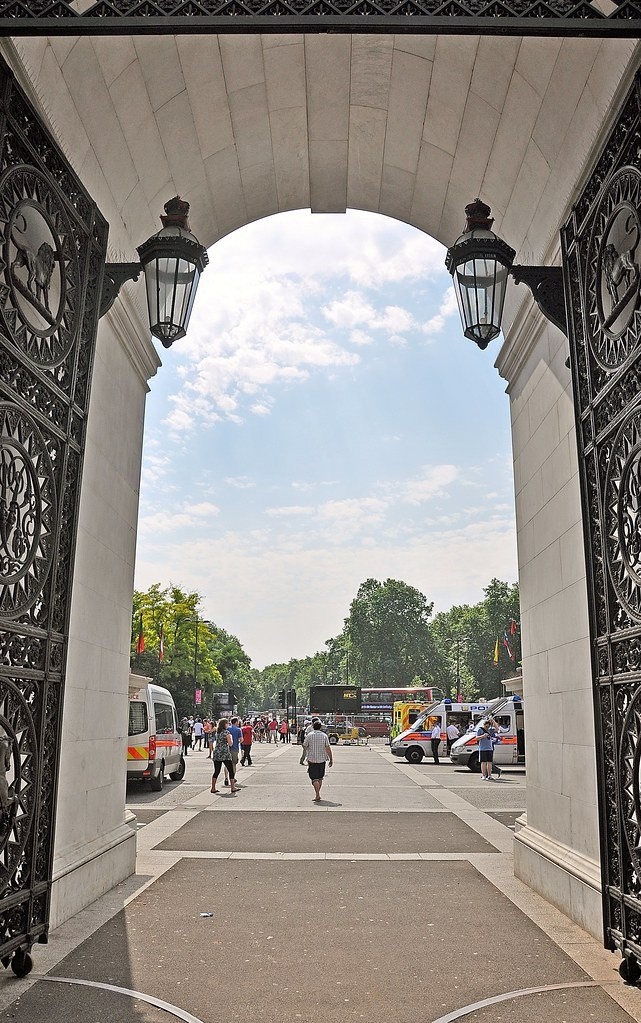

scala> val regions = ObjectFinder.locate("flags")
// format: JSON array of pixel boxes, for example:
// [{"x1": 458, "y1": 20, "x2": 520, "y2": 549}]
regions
[
  {"x1": 504, "y1": 632, "x2": 515, "y2": 662},
  {"x1": 494, "y1": 636, "x2": 499, "y2": 668},
  {"x1": 159, "y1": 634, "x2": 164, "y2": 661},
  {"x1": 511, "y1": 621, "x2": 516, "y2": 635},
  {"x1": 137, "y1": 629, "x2": 144, "y2": 654}
]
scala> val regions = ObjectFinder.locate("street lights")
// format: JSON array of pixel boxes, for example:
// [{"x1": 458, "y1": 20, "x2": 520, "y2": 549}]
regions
[
  {"x1": 444, "y1": 637, "x2": 473, "y2": 703},
  {"x1": 184, "y1": 613, "x2": 211, "y2": 714}
]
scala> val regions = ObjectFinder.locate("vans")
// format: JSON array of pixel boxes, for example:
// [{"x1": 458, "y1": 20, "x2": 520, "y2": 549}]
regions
[{"x1": 126, "y1": 683, "x2": 186, "y2": 791}]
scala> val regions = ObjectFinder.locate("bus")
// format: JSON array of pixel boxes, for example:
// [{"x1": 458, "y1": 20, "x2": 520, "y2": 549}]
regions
[{"x1": 313, "y1": 687, "x2": 445, "y2": 737}]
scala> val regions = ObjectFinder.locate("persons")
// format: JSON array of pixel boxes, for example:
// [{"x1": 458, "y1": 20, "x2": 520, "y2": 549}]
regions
[{"x1": 178, "y1": 713, "x2": 501, "y2": 802}]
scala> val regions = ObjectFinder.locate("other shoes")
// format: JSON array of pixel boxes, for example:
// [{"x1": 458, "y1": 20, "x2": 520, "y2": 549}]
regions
[
  {"x1": 247, "y1": 762, "x2": 252, "y2": 765},
  {"x1": 240, "y1": 760, "x2": 245, "y2": 766},
  {"x1": 225, "y1": 778, "x2": 228, "y2": 786},
  {"x1": 488, "y1": 776, "x2": 496, "y2": 781},
  {"x1": 481, "y1": 775, "x2": 485, "y2": 780}
]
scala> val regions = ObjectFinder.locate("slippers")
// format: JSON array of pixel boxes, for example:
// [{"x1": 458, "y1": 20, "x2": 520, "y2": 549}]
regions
[
  {"x1": 210, "y1": 790, "x2": 219, "y2": 793},
  {"x1": 312, "y1": 795, "x2": 321, "y2": 802},
  {"x1": 231, "y1": 788, "x2": 240, "y2": 792}
]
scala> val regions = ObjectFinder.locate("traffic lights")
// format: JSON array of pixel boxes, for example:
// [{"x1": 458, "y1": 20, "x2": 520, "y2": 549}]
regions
[{"x1": 278, "y1": 689, "x2": 285, "y2": 704}]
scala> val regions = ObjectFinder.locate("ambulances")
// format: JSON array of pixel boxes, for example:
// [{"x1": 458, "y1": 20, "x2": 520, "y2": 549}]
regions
[
  {"x1": 385, "y1": 699, "x2": 437, "y2": 746},
  {"x1": 389, "y1": 699, "x2": 495, "y2": 763},
  {"x1": 449, "y1": 695, "x2": 525, "y2": 772}
]
